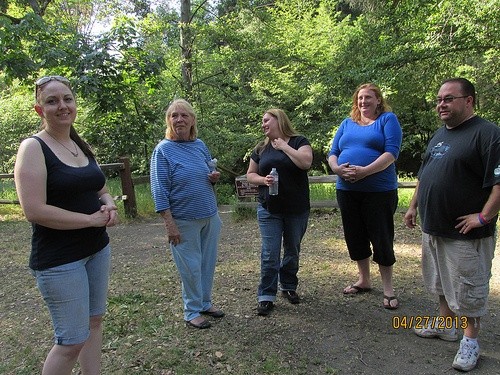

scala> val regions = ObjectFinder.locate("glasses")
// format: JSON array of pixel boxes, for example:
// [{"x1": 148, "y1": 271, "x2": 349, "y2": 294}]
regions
[
  {"x1": 433, "y1": 95, "x2": 475, "y2": 105},
  {"x1": 34, "y1": 76, "x2": 70, "y2": 103}
]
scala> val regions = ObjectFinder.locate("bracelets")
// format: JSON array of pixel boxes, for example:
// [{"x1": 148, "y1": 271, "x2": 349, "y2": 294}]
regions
[{"x1": 479, "y1": 212, "x2": 490, "y2": 225}]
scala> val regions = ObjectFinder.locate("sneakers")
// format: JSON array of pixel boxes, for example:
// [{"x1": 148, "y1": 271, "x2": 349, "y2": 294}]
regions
[
  {"x1": 453, "y1": 340, "x2": 480, "y2": 371},
  {"x1": 414, "y1": 323, "x2": 458, "y2": 343}
]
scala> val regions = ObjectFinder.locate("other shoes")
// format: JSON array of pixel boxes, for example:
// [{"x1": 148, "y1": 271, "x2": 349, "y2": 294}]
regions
[
  {"x1": 187, "y1": 318, "x2": 212, "y2": 329},
  {"x1": 257, "y1": 301, "x2": 273, "y2": 315},
  {"x1": 202, "y1": 307, "x2": 224, "y2": 317},
  {"x1": 283, "y1": 290, "x2": 299, "y2": 303}
]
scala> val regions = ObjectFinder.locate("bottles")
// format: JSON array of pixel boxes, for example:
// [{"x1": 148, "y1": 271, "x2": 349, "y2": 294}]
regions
[
  {"x1": 207, "y1": 158, "x2": 217, "y2": 170},
  {"x1": 269, "y1": 168, "x2": 278, "y2": 195}
]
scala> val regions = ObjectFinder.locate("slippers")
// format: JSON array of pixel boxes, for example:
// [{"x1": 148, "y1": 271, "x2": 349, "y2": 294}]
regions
[
  {"x1": 383, "y1": 294, "x2": 400, "y2": 309},
  {"x1": 343, "y1": 284, "x2": 372, "y2": 294}
]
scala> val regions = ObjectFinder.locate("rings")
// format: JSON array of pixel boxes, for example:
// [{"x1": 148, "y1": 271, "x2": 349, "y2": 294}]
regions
[{"x1": 465, "y1": 224, "x2": 467, "y2": 228}]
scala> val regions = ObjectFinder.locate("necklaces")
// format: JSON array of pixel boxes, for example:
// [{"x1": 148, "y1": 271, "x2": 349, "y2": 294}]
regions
[{"x1": 44, "y1": 128, "x2": 79, "y2": 157}]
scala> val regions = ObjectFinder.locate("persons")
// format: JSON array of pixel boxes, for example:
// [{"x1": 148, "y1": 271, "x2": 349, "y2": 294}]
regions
[
  {"x1": 404, "y1": 79, "x2": 500, "y2": 370},
  {"x1": 14, "y1": 75, "x2": 119, "y2": 375},
  {"x1": 328, "y1": 83, "x2": 401, "y2": 309},
  {"x1": 247, "y1": 108, "x2": 313, "y2": 315},
  {"x1": 150, "y1": 99, "x2": 225, "y2": 329}
]
